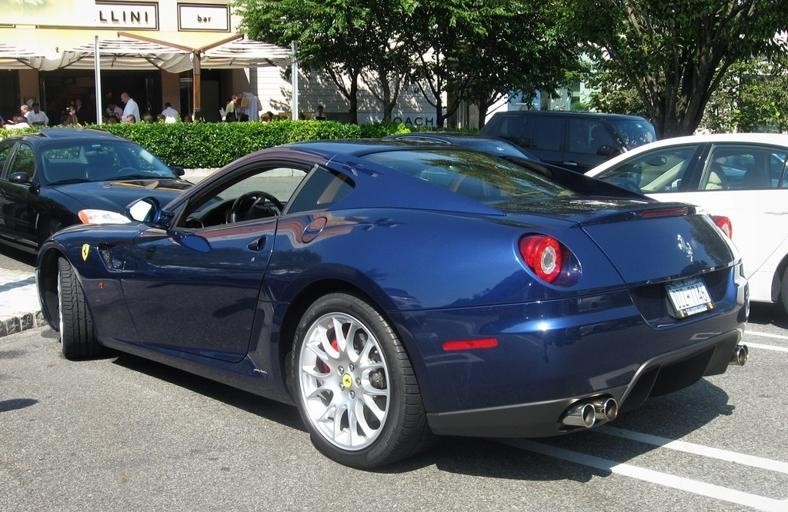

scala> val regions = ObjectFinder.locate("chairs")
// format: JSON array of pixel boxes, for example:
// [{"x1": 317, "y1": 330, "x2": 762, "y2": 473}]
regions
[
  {"x1": 704, "y1": 164, "x2": 725, "y2": 189},
  {"x1": 86, "y1": 150, "x2": 114, "y2": 179}
]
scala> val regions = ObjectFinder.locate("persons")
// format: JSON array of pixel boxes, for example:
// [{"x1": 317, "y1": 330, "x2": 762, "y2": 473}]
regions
[
  {"x1": 315, "y1": 106, "x2": 328, "y2": 121},
  {"x1": 223, "y1": 92, "x2": 273, "y2": 122},
  {"x1": 106, "y1": 91, "x2": 192, "y2": 123},
  {"x1": 12, "y1": 98, "x2": 83, "y2": 126}
]
